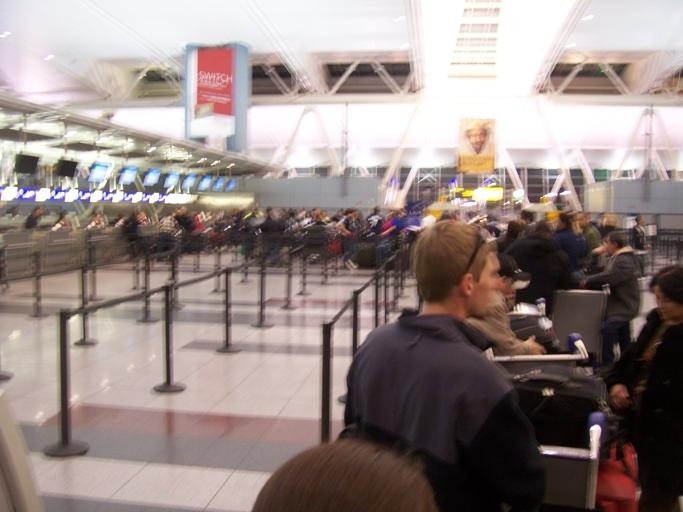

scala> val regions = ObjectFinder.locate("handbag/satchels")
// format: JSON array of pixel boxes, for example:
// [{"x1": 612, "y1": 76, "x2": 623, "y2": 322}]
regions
[{"x1": 597, "y1": 442, "x2": 641, "y2": 512}]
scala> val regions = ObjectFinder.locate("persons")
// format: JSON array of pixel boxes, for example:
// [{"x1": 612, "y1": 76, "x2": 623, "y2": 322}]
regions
[
  {"x1": 460, "y1": 117, "x2": 496, "y2": 155},
  {"x1": 470, "y1": 201, "x2": 651, "y2": 373},
  {"x1": 1, "y1": 201, "x2": 248, "y2": 281},
  {"x1": 601, "y1": 267, "x2": 683, "y2": 511},
  {"x1": 254, "y1": 441, "x2": 437, "y2": 512},
  {"x1": 342, "y1": 220, "x2": 549, "y2": 511},
  {"x1": 249, "y1": 204, "x2": 457, "y2": 270}
]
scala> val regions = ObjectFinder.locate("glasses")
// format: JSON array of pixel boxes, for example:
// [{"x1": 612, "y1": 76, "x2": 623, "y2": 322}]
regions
[{"x1": 465, "y1": 223, "x2": 491, "y2": 273}]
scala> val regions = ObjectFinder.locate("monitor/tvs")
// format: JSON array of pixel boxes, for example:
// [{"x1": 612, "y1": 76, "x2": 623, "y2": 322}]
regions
[
  {"x1": 56, "y1": 160, "x2": 77, "y2": 178},
  {"x1": 117, "y1": 166, "x2": 137, "y2": 185},
  {"x1": 181, "y1": 175, "x2": 237, "y2": 193},
  {"x1": 87, "y1": 162, "x2": 109, "y2": 184},
  {"x1": 15, "y1": 154, "x2": 39, "y2": 174},
  {"x1": 163, "y1": 174, "x2": 180, "y2": 189},
  {"x1": 143, "y1": 168, "x2": 161, "y2": 186}
]
